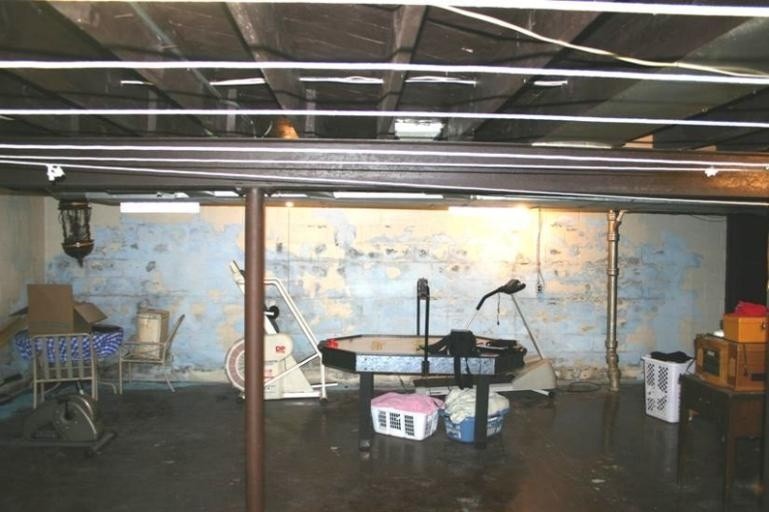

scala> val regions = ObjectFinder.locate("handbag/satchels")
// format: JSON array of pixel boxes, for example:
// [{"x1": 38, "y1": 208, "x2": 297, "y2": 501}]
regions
[{"x1": 448, "y1": 330, "x2": 476, "y2": 356}]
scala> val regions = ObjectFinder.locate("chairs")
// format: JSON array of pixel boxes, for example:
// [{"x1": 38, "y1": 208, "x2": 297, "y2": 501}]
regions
[
  {"x1": 32, "y1": 332, "x2": 99, "y2": 410},
  {"x1": 117, "y1": 313, "x2": 186, "y2": 395}
]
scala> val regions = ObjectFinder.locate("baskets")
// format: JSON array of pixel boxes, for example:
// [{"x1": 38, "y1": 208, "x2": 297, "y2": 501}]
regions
[
  {"x1": 643, "y1": 356, "x2": 696, "y2": 423},
  {"x1": 371, "y1": 404, "x2": 440, "y2": 441}
]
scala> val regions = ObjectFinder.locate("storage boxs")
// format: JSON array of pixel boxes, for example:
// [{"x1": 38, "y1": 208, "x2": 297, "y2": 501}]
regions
[
  {"x1": 8, "y1": 283, "x2": 109, "y2": 337},
  {"x1": 694, "y1": 310, "x2": 768, "y2": 390}
]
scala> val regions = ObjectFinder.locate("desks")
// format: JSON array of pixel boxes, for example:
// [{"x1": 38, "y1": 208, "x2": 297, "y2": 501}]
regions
[
  {"x1": 317, "y1": 334, "x2": 527, "y2": 450},
  {"x1": 14, "y1": 325, "x2": 124, "y2": 402},
  {"x1": 677, "y1": 373, "x2": 769, "y2": 511}
]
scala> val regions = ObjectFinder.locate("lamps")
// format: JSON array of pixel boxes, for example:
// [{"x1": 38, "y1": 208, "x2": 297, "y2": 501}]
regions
[{"x1": 57, "y1": 197, "x2": 94, "y2": 268}]
